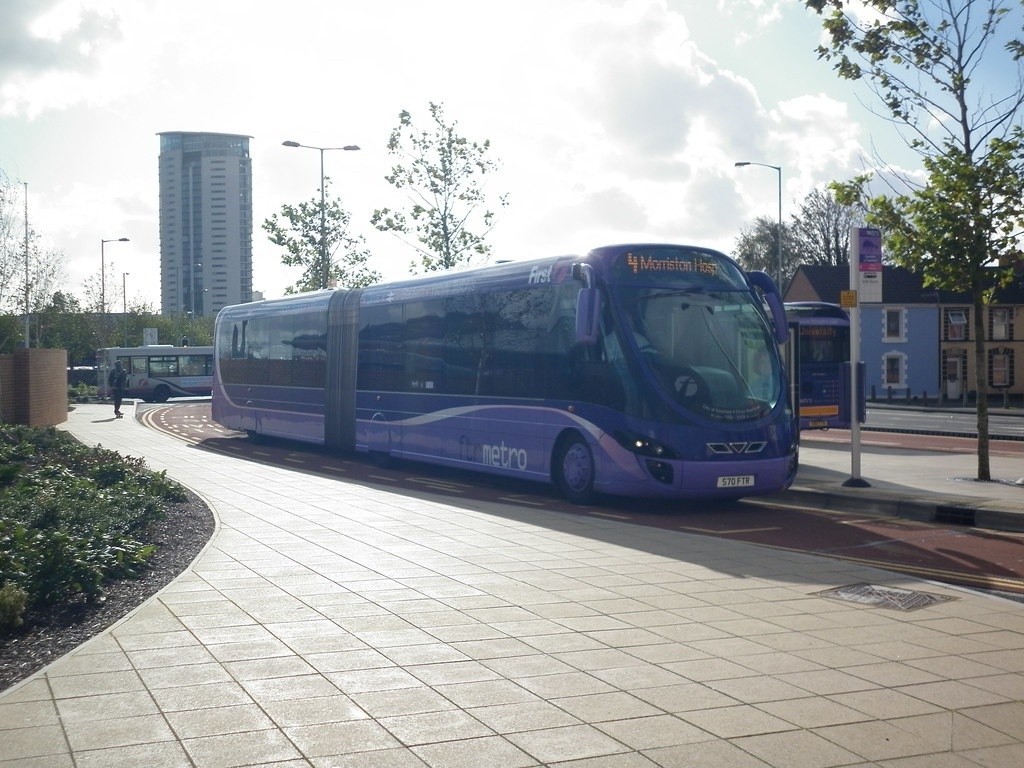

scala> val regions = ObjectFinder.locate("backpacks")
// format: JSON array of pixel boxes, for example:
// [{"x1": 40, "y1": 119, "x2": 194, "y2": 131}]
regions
[{"x1": 110, "y1": 368, "x2": 126, "y2": 390}]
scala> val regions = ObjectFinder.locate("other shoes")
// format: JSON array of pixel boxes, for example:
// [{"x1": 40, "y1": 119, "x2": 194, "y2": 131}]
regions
[{"x1": 114, "y1": 411, "x2": 124, "y2": 415}]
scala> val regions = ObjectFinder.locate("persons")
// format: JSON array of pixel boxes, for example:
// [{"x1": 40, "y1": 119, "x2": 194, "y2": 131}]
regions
[{"x1": 108, "y1": 360, "x2": 128, "y2": 418}]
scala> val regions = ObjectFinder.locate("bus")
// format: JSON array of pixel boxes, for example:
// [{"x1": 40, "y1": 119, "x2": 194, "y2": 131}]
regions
[
  {"x1": 714, "y1": 302, "x2": 852, "y2": 431},
  {"x1": 97, "y1": 344, "x2": 261, "y2": 403},
  {"x1": 212, "y1": 243, "x2": 800, "y2": 504}
]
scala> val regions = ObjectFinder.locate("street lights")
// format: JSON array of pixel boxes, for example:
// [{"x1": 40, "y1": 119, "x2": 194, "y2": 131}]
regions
[
  {"x1": 735, "y1": 162, "x2": 782, "y2": 297},
  {"x1": 191, "y1": 289, "x2": 208, "y2": 346},
  {"x1": 282, "y1": 141, "x2": 360, "y2": 288},
  {"x1": 123, "y1": 272, "x2": 129, "y2": 347},
  {"x1": 175, "y1": 263, "x2": 203, "y2": 346},
  {"x1": 101, "y1": 238, "x2": 131, "y2": 347}
]
